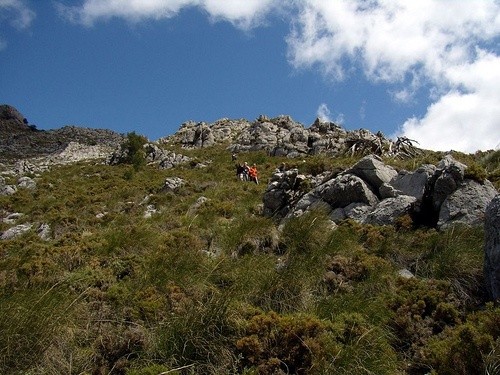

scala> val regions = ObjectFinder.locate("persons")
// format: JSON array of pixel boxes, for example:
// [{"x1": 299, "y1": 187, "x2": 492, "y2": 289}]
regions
[
  {"x1": 237, "y1": 162, "x2": 245, "y2": 182},
  {"x1": 242, "y1": 162, "x2": 251, "y2": 181},
  {"x1": 249, "y1": 163, "x2": 260, "y2": 186}
]
